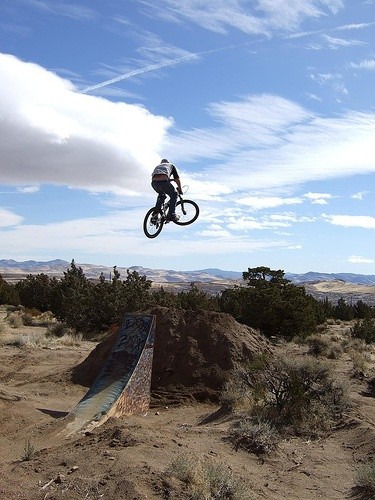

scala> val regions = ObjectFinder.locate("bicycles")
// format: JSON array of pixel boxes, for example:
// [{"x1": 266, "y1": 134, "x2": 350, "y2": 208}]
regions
[{"x1": 143, "y1": 183, "x2": 200, "y2": 239}]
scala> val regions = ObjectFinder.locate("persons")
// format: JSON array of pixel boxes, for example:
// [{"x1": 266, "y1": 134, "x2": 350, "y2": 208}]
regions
[{"x1": 151, "y1": 158, "x2": 183, "y2": 221}]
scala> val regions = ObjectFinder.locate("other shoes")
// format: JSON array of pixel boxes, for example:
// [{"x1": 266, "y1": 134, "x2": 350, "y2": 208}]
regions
[
  {"x1": 167, "y1": 212, "x2": 179, "y2": 221},
  {"x1": 151, "y1": 217, "x2": 158, "y2": 224}
]
